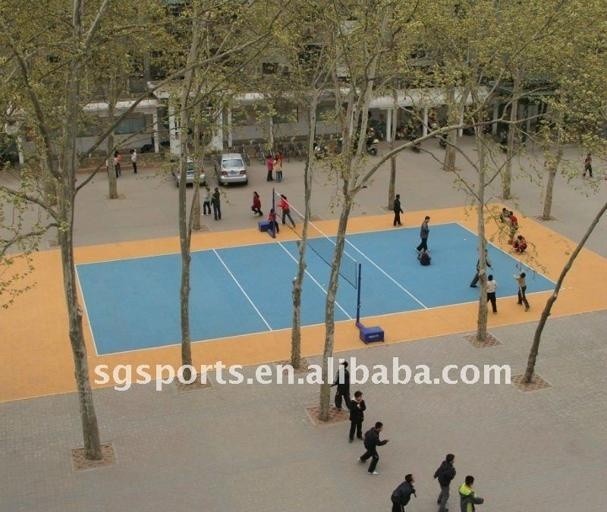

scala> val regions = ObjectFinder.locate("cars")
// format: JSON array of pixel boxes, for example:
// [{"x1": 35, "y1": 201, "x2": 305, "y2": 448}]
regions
[{"x1": 167, "y1": 156, "x2": 209, "y2": 187}]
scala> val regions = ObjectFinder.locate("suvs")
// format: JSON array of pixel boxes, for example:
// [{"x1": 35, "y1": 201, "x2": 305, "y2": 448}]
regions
[{"x1": 211, "y1": 153, "x2": 248, "y2": 187}]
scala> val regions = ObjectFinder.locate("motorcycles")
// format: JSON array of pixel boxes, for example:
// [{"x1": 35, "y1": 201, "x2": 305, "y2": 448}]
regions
[{"x1": 312, "y1": 117, "x2": 487, "y2": 166}]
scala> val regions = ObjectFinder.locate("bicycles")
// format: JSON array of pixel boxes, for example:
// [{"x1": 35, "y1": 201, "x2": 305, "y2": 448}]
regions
[{"x1": 231, "y1": 138, "x2": 309, "y2": 166}]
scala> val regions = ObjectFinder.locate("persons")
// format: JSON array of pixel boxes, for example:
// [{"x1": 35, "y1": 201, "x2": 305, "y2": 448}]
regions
[
  {"x1": 114, "y1": 155, "x2": 119, "y2": 178},
  {"x1": 330, "y1": 360, "x2": 484, "y2": 512},
  {"x1": 583, "y1": 153, "x2": 593, "y2": 177},
  {"x1": 393, "y1": 194, "x2": 403, "y2": 227},
  {"x1": 129, "y1": 149, "x2": 137, "y2": 174},
  {"x1": 202, "y1": 154, "x2": 297, "y2": 234},
  {"x1": 415, "y1": 215, "x2": 432, "y2": 252},
  {"x1": 106, "y1": 159, "x2": 109, "y2": 176},
  {"x1": 469, "y1": 205, "x2": 531, "y2": 312},
  {"x1": 115, "y1": 151, "x2": 121, "y2": 176}
]
